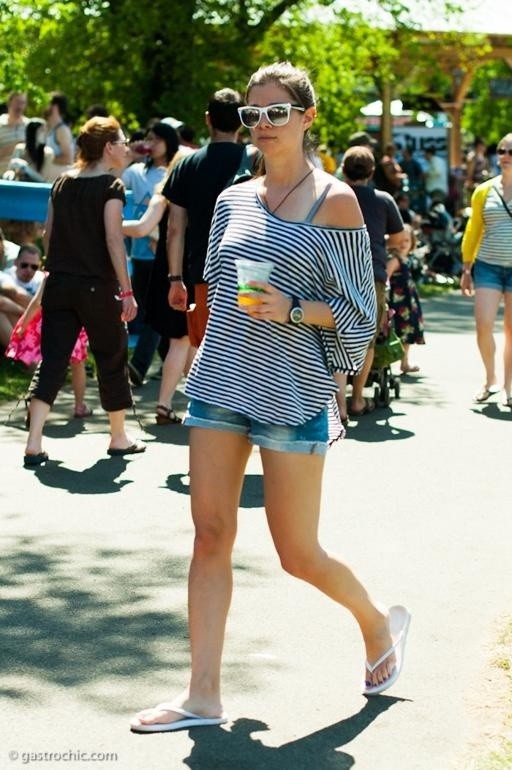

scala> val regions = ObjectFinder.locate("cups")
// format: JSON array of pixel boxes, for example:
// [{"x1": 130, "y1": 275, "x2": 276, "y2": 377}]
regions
[
  {"x1": 132, "y1": 141, "x2": 153, "y2": 156},
  {"x1": 233, "y1": 259, "x2": 274, "y2": 310}
]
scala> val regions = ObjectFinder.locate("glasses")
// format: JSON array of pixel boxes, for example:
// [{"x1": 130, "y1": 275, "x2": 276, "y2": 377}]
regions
[
  {"x1": 237, "y1": 103, "x2": 305, "y2": 128},
  {"x1": 21, "y1": 262, "x2": 38, "y2": 270},
  {"x1": 498, "y1": 150, "x2": 512, "y2": 155}
]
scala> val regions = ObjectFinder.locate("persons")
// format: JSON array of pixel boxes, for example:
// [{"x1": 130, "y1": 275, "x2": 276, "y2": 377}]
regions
[
  {"x1": 22, "y1": 112, "x2": 143, "y2": 467},
  {"x1": 129, "y1": 60, "x2": 413, "y2": 739},
  {"x1": 0, "y1": 86, "x2": 512, "y2": 427}
]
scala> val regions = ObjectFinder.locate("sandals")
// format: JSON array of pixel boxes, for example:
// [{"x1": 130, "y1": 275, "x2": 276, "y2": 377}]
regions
[
  {"x1": 156, "y1": 405, "x2": 182, "y2": 424},
  {"x1": 75, "y1": 404, "x2": 92, "y2": 416}
]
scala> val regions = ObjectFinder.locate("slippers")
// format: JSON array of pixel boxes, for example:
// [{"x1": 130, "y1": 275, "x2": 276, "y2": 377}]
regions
[
  {"x1": 131, "y1": 706, "x2": 226, "y2": 731},
  {"x1": 108, "y1": 444, "x2": 145, "y2": 454},
  {"x1": 351, "y1": 399, "x2": 375, "y2": 416},
  {"x1": 24, "y1": 451, "x2": 48, "y2": 463},
  {"x1": 364, "y1": 606, "x2": 410, "y2": 696},
  {"x1": 477, "y1": 389, "x2": 512, "y2": 406}
]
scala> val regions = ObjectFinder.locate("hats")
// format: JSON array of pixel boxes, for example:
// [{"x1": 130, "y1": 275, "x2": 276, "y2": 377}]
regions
[
  {"x1": 161, "y1": 117, "x2": 183, "y2": 131},
  {"x1": 349, "y1": 133, "x2": 377, "y2": 145}
]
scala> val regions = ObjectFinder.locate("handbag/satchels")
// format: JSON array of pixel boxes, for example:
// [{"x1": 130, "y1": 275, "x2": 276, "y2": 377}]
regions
[{"x1": 373, "y1": 339, "x2": 405, "y2": 370}]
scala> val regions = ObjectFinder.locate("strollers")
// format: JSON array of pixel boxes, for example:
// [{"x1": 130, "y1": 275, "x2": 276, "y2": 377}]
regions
[
  {"x1": 418, "y1": 202, "x2": 471, "y2": 287},
  {"x1": 344, "y1": 234, "x2": 407, "y2": 409}
]
